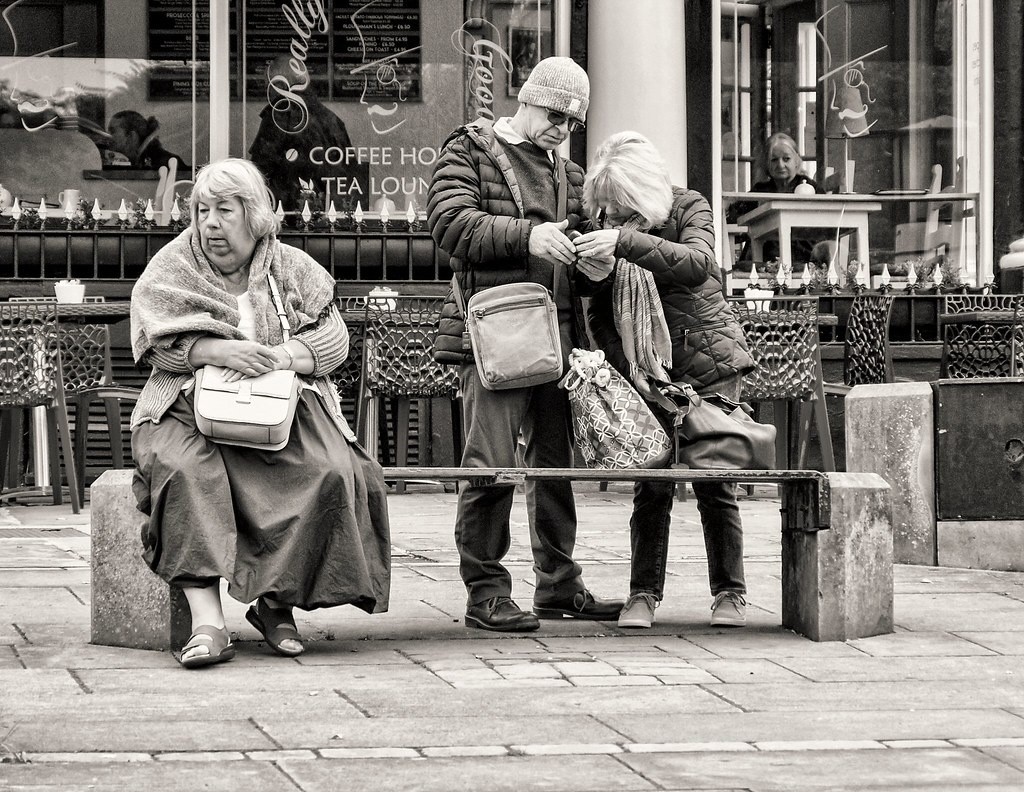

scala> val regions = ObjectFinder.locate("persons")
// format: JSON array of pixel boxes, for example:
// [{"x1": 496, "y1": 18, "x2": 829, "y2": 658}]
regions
[
  {"x1": 108, "y1": 110, "x2": 189, "y2": 171},
  {"x1": 573, "y1": 130, "x2": 755, "y2": 627},
  {"x1": 130, "y1": 158, "x2": 390, "y2": 666},
  {"x1": 248, "y1": 55, "x2": 362, "y2": 228},
  {"x1": 425, "y1": 57, "x2": 628, "y2": 632},
  {"x1": 730, "y1": 132, "x2": 833, "y2": 263}
]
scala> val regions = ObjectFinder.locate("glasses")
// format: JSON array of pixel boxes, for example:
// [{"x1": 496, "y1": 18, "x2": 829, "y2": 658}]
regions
[
  {"x1": 545, "y1": 108, "x2": 586, "y2": 134},
  {"x1": 108, "y1": 125, "x2": 123, "y2": 134}
]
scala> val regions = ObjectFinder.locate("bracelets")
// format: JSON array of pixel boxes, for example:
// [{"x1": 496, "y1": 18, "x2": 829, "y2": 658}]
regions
[{"x1": 281, "y1": 345, "x2": 293, "y2": 369}]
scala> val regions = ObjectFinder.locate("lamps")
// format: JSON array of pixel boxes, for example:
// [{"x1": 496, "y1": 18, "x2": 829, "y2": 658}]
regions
[{"x1": 823, "y1": 86, "x2": 865, "y2": 193}]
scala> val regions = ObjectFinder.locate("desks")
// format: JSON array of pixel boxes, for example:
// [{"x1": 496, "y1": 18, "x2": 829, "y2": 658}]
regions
[
  {"x1": 0, "y1": 302, "x2": 130, "y2": 324},
  {"x1": 731, "y1": 310, "x2": 837, "y2": 471},
  {"x1": 339, "y1": 309, "x2": 442, "y2": 463},
  {"x1": 938, "y1": 311, "x2": 1024, "y2": 380},
  {"x1": 737, "y1": 200, "x2": 882, "y2": 288}
]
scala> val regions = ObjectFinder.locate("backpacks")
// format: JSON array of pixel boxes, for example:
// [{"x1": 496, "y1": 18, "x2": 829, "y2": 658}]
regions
[{"x1": 558, "y1": 347, "x2": 672, "y2": 469}]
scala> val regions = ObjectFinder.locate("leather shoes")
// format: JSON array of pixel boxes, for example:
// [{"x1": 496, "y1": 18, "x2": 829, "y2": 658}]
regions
[
  {"x1": 533, "y1": 589, "x2": 625, "y2": 621},
  {"x1": 465, "y1": 598, "x2": 540, "y2": 631}
]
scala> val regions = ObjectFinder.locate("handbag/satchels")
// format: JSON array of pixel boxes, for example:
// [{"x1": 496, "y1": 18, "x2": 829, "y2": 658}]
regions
[
  {"x1": 650, "y1": 379, "x2": 777, "y2": 470},
  {"x1": 462, "y1": 282, "x2": 563, "y2": 390},
  {"x1": 194, "y1": 365, "x2": 303, "y2": 451}
]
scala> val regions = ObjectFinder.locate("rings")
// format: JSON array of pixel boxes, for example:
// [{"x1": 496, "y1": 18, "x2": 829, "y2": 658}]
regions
[
  {"x1": 592, "y1": 250, "x2": 595, "y2": 256},
  {"x1": 560, "y1": 236, "x2": 564, "y2": 242}
]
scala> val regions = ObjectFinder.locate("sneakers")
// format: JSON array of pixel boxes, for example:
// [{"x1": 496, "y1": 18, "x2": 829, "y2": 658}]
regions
[
  {"x1": 617, "y1": 592, "x2": 660, "y2": 628},
  {"x1": 711, "y1": 591, "x2": 747, "y2": 626}
]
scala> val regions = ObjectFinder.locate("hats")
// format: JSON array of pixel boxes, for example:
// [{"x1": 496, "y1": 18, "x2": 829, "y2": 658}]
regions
[{"x1": 517, "y1": 56, "x2": 590, "y2": 122}]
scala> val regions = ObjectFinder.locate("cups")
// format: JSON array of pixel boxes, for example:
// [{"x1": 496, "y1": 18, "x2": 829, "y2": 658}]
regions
[{"x1": 58, "y1": 190, "x2": 81, "y2": 211}]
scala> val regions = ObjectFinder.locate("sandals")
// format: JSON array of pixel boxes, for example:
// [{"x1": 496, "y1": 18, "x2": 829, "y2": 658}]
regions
[
  {"x1": 180, "y1": 625, "x2": 235, "y2": 668},
  {"x1": 245, "y1": 596, "x2": 305, "y2": 656}
]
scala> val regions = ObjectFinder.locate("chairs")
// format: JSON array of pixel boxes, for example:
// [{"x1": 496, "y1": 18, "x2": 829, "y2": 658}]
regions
[
  {"x1": 325, "y1": 293, "x2": 463, "y2": 490},
  {"x1": 722, "y1": 293, "x2": 1024, "y2": 477},
  {"x1": 804, "y1": 161, "x2": 969, "y2": 276},
  {"x1": 2, "y1": 292, "x2": 147, "y2": 513}
]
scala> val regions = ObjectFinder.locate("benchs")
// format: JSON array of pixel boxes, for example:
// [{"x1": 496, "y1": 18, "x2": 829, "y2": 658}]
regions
[{"x1": 88, "y1": 464, "x2": 892, "y2": 645}]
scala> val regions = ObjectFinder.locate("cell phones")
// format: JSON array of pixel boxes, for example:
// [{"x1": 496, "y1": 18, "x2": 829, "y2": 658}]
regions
[{"x1": 568, "y1": 230, "x2": 586, "y2": 259}]
